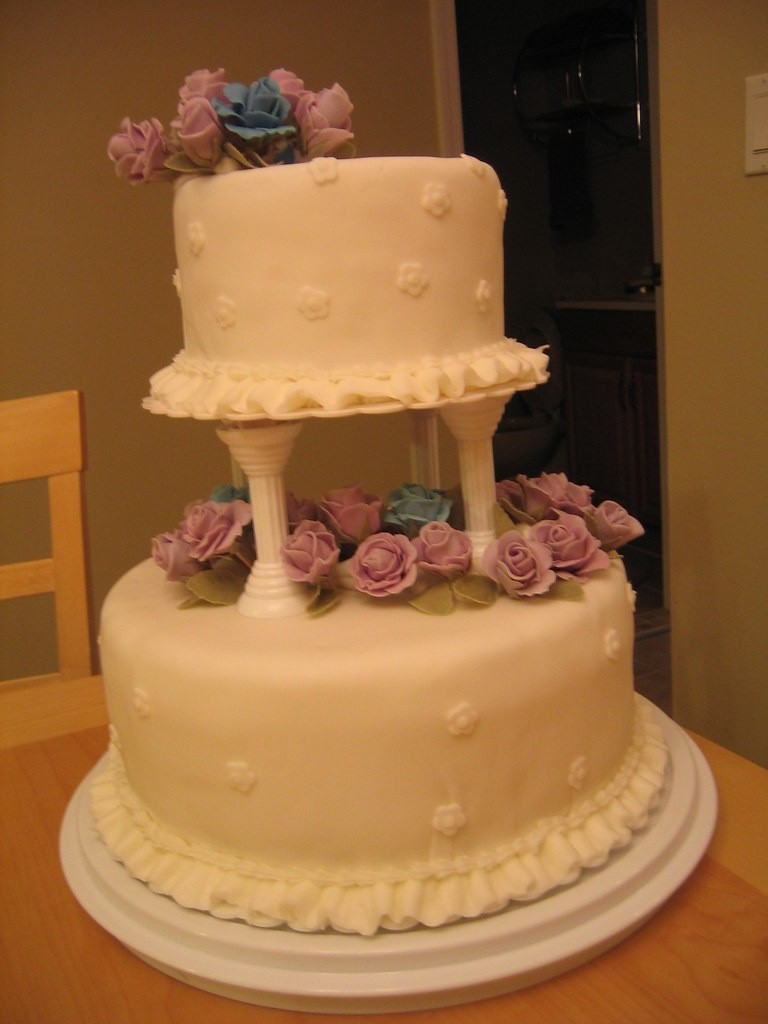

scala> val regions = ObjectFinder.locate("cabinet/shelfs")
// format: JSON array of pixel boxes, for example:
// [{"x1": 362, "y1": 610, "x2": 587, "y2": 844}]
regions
[{"x1": 557, "y1": 309, "x2": 661, "y2": 560}]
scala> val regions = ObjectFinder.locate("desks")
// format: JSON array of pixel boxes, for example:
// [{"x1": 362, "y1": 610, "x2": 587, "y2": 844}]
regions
[{"x1": 0, "y1": 672, "x2": 768, "y2": 1024}]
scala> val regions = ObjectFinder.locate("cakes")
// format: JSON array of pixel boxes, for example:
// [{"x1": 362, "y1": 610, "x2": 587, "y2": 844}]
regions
[{"x1": 89, "y1": 66, "x2": 672, "y2": 934}]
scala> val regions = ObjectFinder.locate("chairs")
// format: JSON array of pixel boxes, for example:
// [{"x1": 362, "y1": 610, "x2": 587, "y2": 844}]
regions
[{"x1": 0, "y1": 389, "x2": 91, "y2": 696}]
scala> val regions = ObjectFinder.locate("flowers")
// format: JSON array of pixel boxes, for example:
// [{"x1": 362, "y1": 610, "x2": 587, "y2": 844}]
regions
[
  {"x1": 108, "y1": 68, "x2": 355, "y2": 188},
  {"x1": 149, "y1": 468, "x2": 646, "y2": 619}
]
